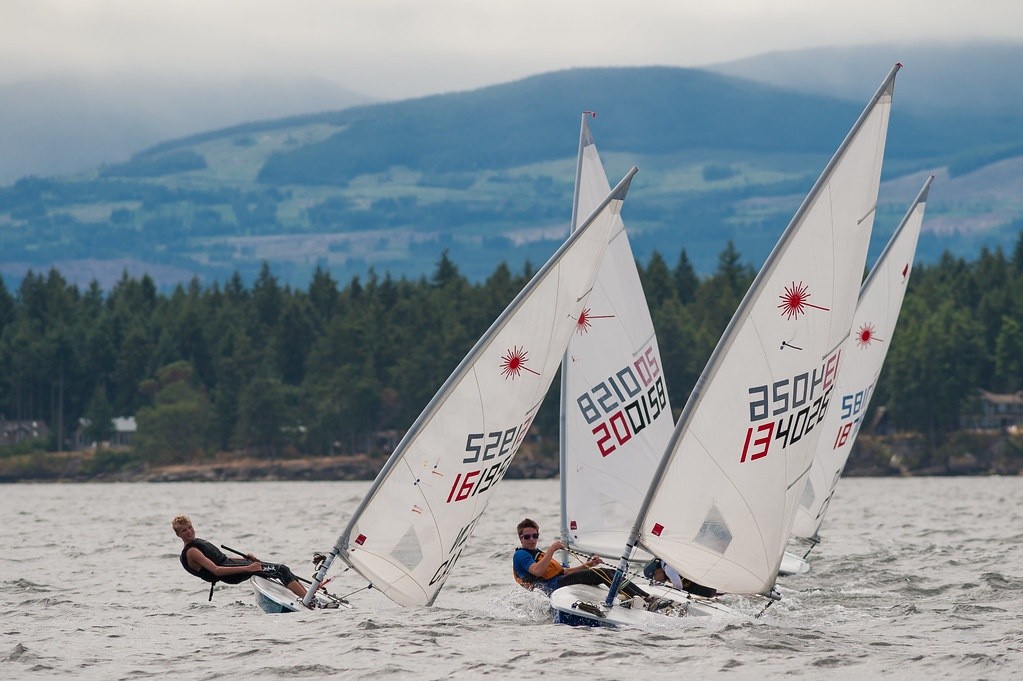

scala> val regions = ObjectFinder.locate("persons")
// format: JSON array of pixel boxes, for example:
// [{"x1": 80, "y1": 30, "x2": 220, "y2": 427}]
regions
[
  {"x1": 170, "y1": 515, "x2": 309, "y2": 597},
  {"x1": 654, "y1": 556, "x2": 716, "y2": 598},
  {"x1": 513, "y1": 519, "x2": 674, "y2": 612}
]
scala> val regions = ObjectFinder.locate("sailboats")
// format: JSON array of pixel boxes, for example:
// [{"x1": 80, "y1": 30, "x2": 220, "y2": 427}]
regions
[
  {"x1": 249, "y1": 162, "x2": 641, "y2": 621},
  {"x1": 547, "y1": 54, "x2": 939, "y2": 639}
]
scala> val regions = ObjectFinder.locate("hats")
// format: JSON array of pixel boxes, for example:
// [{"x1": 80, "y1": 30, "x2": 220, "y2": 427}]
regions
[{"x1": 644, "y1": 560, "x2": 659, "y2": 580}]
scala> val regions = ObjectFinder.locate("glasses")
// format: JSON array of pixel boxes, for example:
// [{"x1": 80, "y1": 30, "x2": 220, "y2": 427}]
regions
[{"x1": 521, "y1": 533, "x2": 540, "y2": 540}]
